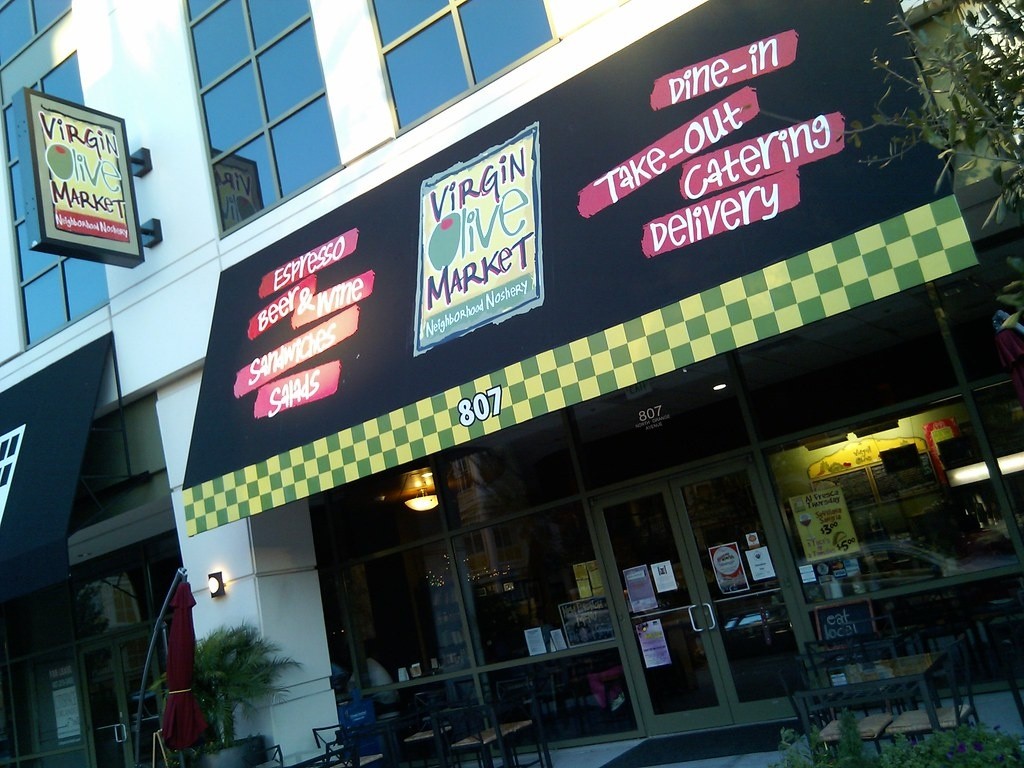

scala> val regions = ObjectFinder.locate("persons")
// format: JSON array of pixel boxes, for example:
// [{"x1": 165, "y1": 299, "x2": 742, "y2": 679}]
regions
[{"x1": 346, "y1": 647, "x2": 398, "y2": 714}]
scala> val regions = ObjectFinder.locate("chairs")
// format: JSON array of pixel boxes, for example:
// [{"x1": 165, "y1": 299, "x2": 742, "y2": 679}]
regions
[
  {"x1": 476, "y1": 704, "x2": 534, "y2": 758},
  {"x1": 406, "y1": 707, "x2": 455, "y2": 762},
  {"x1": 437, "y1": 711, "x2": 502, "y2": 764},
  {"x1": 245, "y1": 745, "x2": 287, "y2": 768},
  {"x1": 886, "y1": 634, "x2": 972, "y2": 759},
  {"x1": 315, "y1": 725, "x2": 345, "y2": 751},
  {"x1": 783, "y1": 668, "x2": 896, "y2": 757},
  {"x1": 327, "y1": 736, "x2": 384, "y2": 768}
]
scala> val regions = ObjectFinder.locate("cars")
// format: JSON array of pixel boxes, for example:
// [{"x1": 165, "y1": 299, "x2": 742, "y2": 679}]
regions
[{"x1": 808, "y1": 540, "x2": 1013, "y2": 605}]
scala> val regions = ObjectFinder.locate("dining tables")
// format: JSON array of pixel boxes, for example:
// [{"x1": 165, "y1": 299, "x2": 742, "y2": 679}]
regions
[
  {"x1": 794, "y1": 647, "x2": 951, "y2": 760},
  {"x1": 255, "y1": 742, "x2": 351, "y2": 768},
  {"x1": 450, "y1": 700, "x2": 556, "y2": 767},
  {"x1": 342, "y1": 710, "x2": 421, "y2": 765}
]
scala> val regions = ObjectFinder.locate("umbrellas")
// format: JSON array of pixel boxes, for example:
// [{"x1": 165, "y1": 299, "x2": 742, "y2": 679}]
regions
[{"x1": 160, "y1": 580, "x2": 214, "y2": 753}]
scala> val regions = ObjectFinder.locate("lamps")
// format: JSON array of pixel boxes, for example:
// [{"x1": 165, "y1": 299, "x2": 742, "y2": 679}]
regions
[
  {"x1": 207, "y1": 572, "x2": 226, "y2": 597},
  {"x1": 405, "y1": 487, "x2": 441, "y2": 513}
]
